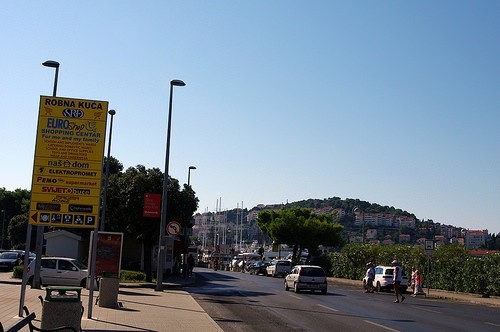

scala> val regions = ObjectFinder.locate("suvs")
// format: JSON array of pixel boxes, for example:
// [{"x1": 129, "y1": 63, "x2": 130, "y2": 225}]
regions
[
  {"x1": 363, "y1": 266, "x2": 408, "y2": 292},
  {"x1": 266, "y1": 260, "x2": 290, "y2": 276}
]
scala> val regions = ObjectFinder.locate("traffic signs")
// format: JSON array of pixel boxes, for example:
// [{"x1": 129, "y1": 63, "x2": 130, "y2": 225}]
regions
[{"x1": 143, "y1": 193, "x2": 160, "y2": 217}]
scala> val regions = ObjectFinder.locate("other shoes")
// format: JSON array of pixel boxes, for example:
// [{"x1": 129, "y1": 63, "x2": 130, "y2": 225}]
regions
[{"x1": 365, "y1": 289, "x2": 374, "y2": 292}]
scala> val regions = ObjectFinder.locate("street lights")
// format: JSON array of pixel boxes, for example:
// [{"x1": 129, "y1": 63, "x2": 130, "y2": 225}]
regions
[
  {"x1": 42, "y1": 59, "x2": 60, "y2": 97},
  {"x1": 188, "y1": 166, "x2": 197, "y2": 188},
  {"x1": 155, "y1": 79, "x2": 186, "y2": 291},
  {"x1": 100, "y1": 110, "x2": 116, "y2": 230}
]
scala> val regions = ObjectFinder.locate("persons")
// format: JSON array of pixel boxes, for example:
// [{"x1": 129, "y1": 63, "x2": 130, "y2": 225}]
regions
[
  {"x1": 391, "y1": 259, "x2": 405, "y2": 303},
  {"x1": 365, "y1": 262, "x2": 375, "y2": 293},
  {"x1": 410, "y1": 267, "x2": 422, "y2": 298},
  {"x1": 187, "y1": 254, "x2": 195, "y2": 278}
]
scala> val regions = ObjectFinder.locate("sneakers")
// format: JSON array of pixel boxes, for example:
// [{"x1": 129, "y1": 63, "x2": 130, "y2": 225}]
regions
[{"x1": 393, "y1": 295, "x2": 405, "y2": 302}]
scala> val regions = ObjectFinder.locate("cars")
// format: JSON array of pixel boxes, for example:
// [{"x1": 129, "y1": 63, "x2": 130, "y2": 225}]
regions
[
  {"x1": 0, "y1": 250, "x2": 36, "y2": 271},
  {"x1": 284, "y1": 266, "x2": 327, "y2": 294},
  {"x1": 25, "y1": 258, "x2": 88, "y2": 287},
  {"x1": 20, "y1": 257, "x2": 34, "y2": 267},
  {"x1": 250, "y1": 262, "x2": 269, "y2": 274}
]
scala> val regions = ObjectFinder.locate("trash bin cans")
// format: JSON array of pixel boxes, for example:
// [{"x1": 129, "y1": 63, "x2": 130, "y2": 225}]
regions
[
  {"x1": 99, "y1": 278, "x2": 120, "y2": 308},
  {"x1": 38, "y1": 286, "x2": 84, "y2": 332}
]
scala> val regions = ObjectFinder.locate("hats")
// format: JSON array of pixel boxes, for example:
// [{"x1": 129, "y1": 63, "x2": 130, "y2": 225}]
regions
[{"x1": 391, "y1": 259, "x2": 397, "y2": 263}]
